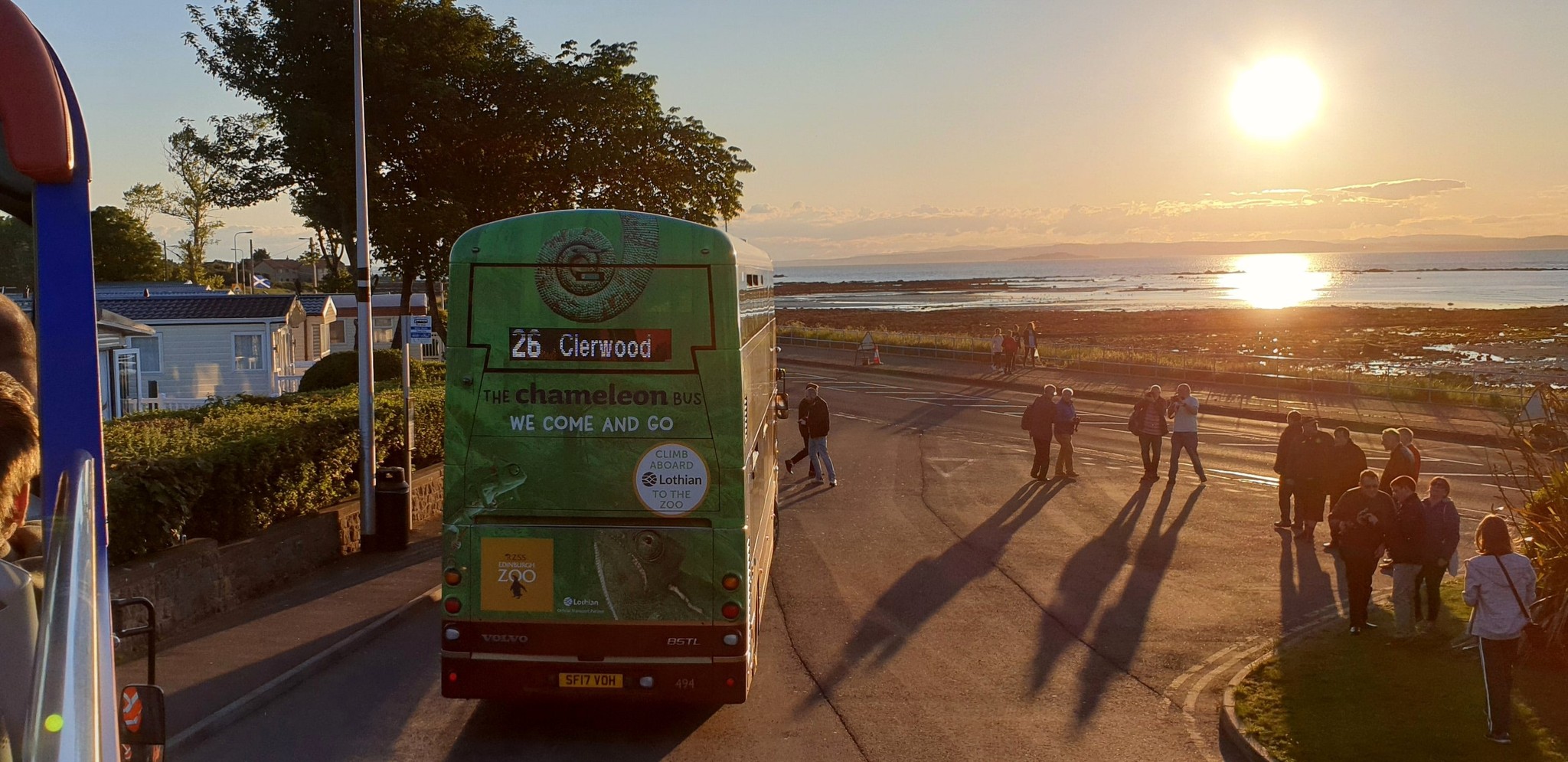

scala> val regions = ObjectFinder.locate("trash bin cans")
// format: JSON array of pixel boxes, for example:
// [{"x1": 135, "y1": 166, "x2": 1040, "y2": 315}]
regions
[{"x1": 375, "y1": 467, "x2": 410, "y2": 551}]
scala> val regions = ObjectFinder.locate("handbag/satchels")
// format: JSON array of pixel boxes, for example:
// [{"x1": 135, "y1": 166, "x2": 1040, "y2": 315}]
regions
[
  {"x1": 1449, "y1": 549, "x2": 1460, "y2": 577},
  {"x1": 1527, "y1": 622, "x2": 1549, "y2": 648},
  {"x1": 1034, "y1": 348, "x2": 1039, "y2": 359}
]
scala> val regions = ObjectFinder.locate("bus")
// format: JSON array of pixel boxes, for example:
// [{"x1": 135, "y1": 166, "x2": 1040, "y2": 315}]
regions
[{"x1": 439, "y1": 208, "x2": 789, "y2": 708}]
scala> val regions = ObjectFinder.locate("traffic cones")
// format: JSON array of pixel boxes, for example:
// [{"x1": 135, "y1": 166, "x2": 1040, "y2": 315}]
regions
[{"x1": 870, "y1": 345, "x2": 883, "y2": 365}]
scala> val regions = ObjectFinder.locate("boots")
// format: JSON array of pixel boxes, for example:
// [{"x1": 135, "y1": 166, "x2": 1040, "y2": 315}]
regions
[{"x1": 1294, "y1": 525, "x2": 1314, "y2": 545}]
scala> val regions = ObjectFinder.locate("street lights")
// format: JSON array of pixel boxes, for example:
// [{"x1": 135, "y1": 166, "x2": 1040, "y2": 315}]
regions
[
  {"x1": 299, "y1": 237, "x2": 318, "y2": 294},
  {"x1": 230, "y1": 248, "x2": 246, "y2": 295},
  {"x1": 234, "y1": 230, "x2": 253, "y2": 294},
  {"x1": 157, "y1": 240, "x2": 168, "y2": 283}
]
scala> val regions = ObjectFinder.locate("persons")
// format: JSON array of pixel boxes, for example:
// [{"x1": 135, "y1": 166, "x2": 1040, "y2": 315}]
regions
[
  {"x1": 1417, "y1": 475, "x2": 1460, "y2": 621},
  {"x1": 0, "y1": 293, "x2": 42, "y2": 762},
  {"x1": 991, "y1": 323, "x2": 1038, "y2": 374},
  {"x1": 1328, "y1": 471, "x2": 1397, "y2": 633},
  {"x1": 1462, "y1": 515, "x2": 1536, "y2": 744},
  {"x1": 1054, "y1": 388, "x2": 1080, "y2": 476},
  {"x1": 785, "y1": 383, "x2": 837, "y2": 486},
  {"x1": 1167, "y1": 383, "x2": 1207, "y2": 484},
  {"x1": 1274, "y1": 411, "x2": 1367, "y2": 547},
  {"x1": 1134, "y1": 385, "x2": 1167, "y2": 481},
  {"x1": 1377, "y1": 428, "x2": 1421, "y2": 570},
  {"x1": 1031, "y1": 385, "x2": 1056, "y2": 481},
  {"x1": 1390, "y1": 475, "x2": 1426, "y2": 639}
]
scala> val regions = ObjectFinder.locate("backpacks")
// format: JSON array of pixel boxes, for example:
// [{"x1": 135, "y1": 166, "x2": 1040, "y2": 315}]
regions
[
  {"x1": 1021, "y1": 396, "x2": 1040, "y2": 430},
  {"x1": 1128, "y1": 410, "x2": 1140, "y2": 435}
]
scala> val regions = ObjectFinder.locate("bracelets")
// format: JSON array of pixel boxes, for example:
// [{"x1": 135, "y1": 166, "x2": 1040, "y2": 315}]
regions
[{"x1": 1183, "y1": 403, "x2": 1186, "y2": 406}]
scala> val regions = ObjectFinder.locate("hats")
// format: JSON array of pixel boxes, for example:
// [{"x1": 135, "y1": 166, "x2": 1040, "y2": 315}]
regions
[{"x1": 1302, "y1": 415, "x2": 1319, "y2": 427}]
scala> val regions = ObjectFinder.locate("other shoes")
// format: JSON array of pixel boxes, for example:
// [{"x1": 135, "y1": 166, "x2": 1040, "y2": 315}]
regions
[
  {"x1": 1066, "y1": 470, "x2": 1079, "y2": 478},
  {"x1": 1379, "y1": 558, "x2": 1393, "y2": 568},
  {"x1": 1036, "y1": 478, "x2": 1052, "y2": 483},
  {"x1": 1484, "y1": 732, "x2": 1512, "y2": 744},
  {"x1": 785, "y1": 460, "x2": 795, "y2": 475},
  {"x1": 1363, "y1": 623, "x2": 1379, "y2": 630},
  {"x1": 1275, "y1": 520, "x2": 1290, "y2": 527},
  {"x1": 1055, "y1": 472, "x2": 1067, "y2": 476},
  {"x1": 1166, "y1": 479, "x2": 1176, "y2": 485},
  {"x1": 1141, "y1": 471, "x2": 1149, "y2": 480},
  {"x1": 1199, "y1": 476, "x2": 1208, "y2": 483},
  {"x1": 1323, "y1": 543, "x2": 1335, "y2": 548},
  {"x1": 1291, "y1": 522, "x2": 1303, "y2": 528},
  {"x1": 811, "y1": 478, "x2": 824, "y2": 484},
  {"x1": 1030, "y1": 474, "x2": 1038, "y2": 480},
  {"x1": 808, "y1": 471, "x2": 824, "y2": 477},
  {"x1": 1350, "y1": 626, "x2": 1362, "y2": 635},
  {"x1": 829, "y1": 478, "x2": 837, "y2": 486}
]
